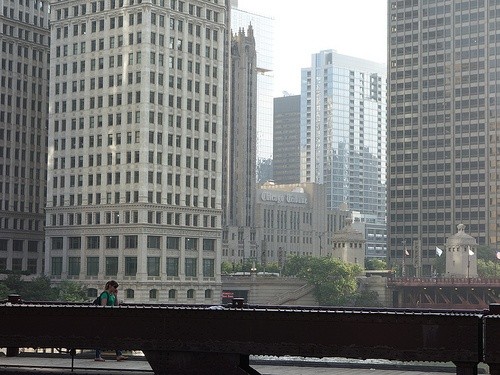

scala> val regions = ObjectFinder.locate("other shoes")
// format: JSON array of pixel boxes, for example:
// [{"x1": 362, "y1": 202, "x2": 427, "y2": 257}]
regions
[{"x1": 95, "y1": 357, "x2": 105, "y2": 362}]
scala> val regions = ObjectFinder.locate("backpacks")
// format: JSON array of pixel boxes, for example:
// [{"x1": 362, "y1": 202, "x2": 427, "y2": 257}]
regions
[{"x1": 91, "y1": 291, "x2": 108, "y2": 308}]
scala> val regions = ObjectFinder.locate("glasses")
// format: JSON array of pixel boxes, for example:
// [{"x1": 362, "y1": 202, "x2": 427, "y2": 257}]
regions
[{"x1": 111, "y1": 284, "x2": 117, "y2": 289}]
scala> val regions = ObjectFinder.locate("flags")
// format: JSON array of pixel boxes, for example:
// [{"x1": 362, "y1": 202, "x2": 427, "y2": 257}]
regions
[
  {"x1": 468, "y1": 248, "x2": 474, "y2": 256},
  {"x1": 436, "y1": 247, "x2": 443, "y2": 257},
  {"x1": 496, "y1": 251, "x2": 500, "y2": 259},
  {"x1": 404, "y1": 248, "x2": 410, "y2": 256}
]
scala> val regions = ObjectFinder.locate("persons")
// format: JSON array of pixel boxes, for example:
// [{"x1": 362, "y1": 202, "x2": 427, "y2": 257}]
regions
[{"x1": 94, "y1": 280, "x2": 130, "y2": 362}]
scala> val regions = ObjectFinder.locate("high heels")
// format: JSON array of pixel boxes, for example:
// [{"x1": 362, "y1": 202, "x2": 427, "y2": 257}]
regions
[{"x1": 117, "y1": 355, "x2": 129, "y2": 361}]
